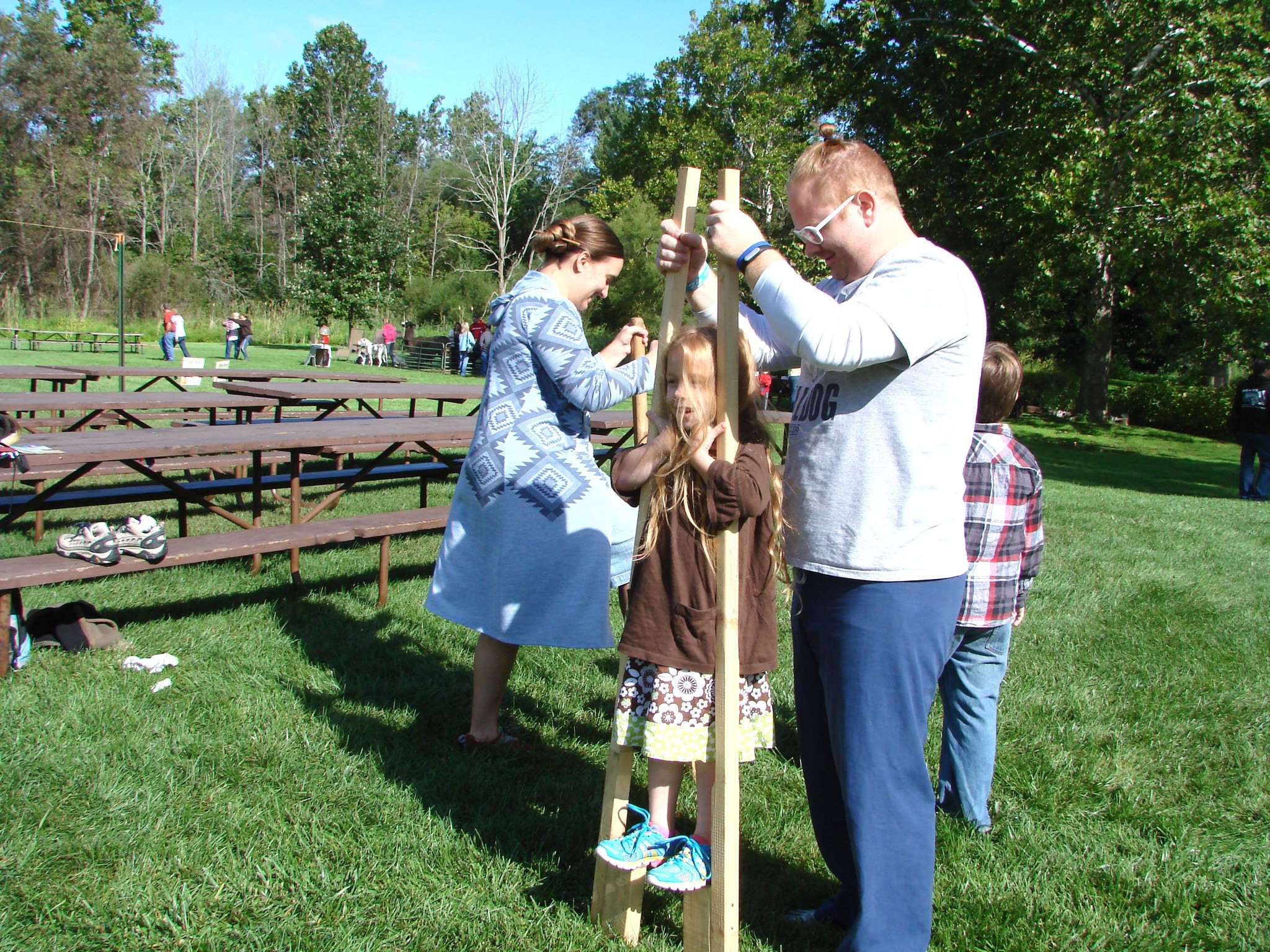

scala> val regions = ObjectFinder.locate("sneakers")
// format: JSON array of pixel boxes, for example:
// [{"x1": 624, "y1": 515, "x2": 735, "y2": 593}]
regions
[
  {"x1": 56, "y1": 514, "x2": 170, "y2": 563},
  {"x1": 645, "y1": 835, "x2": 712, "y2": 892},
  {"x1": 457, "y1": 726, "x2": 534, "y2": 751},
  {"x1": 595, "y1": 804, "x2": 681, "y2": 870}
]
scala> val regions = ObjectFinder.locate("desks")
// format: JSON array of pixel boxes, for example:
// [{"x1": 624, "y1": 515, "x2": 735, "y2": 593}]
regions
[
  {"x1": 87, "y1": 332, "x2": 144, "y2": 354},
  {"x1": 0, "y1": 359, "x2": 795, "y2": 591},
  {"x1": 26, "y1": 330, "x2": 82, "y2": 352},
  {"x1": 0, "y1": 327, "x2": 24, "y2": 350}
]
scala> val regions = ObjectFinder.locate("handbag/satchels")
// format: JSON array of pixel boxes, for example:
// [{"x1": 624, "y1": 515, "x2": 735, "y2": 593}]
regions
[{"x1": 467, "y1": 348, "x2": 474, "y2": 354}]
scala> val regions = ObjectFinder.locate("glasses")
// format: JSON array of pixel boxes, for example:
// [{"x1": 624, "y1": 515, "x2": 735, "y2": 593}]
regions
[{"x1": 793, "y1": 189, "x2": 876, "y2": 244}]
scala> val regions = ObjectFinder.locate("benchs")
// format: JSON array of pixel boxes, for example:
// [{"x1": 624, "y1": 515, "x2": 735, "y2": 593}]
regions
[
  {"x1": 0, "y1": 518, "x2": 355, "y2": 679},
  {"x1": 84, "y1": 341, "x2": 151, "y2": 355},
  {"x1": 28, "y1": 338, "x2": 84, "y2": 352},
  {"x1": 351, "y1": 503, "x2": 453, "y2": 609},
  {"x1": 1, "y1": 337, "x2": 22, "y2": 350}
]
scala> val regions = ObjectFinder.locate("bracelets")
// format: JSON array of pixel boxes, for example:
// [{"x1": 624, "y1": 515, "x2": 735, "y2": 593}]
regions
[
  {"x1": 686, "y1": 262, "x2": 710, "y2": 292},
  {"x1": 737, "y1": 241, "x2": 775, "y2": 274}
]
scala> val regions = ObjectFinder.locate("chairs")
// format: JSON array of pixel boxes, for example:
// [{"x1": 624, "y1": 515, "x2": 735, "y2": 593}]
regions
[{"x1": 337, "y1": 328, "x2": 365, "y2": 363}]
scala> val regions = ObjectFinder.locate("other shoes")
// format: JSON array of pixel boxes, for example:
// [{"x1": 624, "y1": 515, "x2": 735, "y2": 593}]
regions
[{"x1": 1239, "y1": 485, "x2": 1267, "y2": 501}]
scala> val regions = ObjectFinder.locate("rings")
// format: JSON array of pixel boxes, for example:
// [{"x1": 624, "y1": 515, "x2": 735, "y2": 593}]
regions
[{"x1": 706, "y1": 225, "x2": 711, "y2": 238}]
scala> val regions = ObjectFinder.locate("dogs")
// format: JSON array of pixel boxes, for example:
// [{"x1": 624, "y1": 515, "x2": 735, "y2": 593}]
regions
[{"x1": 357, "y1": 338, "x2": 388, "y2": 368}]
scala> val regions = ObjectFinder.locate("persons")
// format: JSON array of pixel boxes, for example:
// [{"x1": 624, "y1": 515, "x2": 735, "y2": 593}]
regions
[
  {"x1": 787, "y1": 368, "x2": 802, "y2": 413},
  {"x1": 221, "y1": 312, "x2": 240, "y2": 360},
  {"x1": 425, "y1": 213, "x2": 662, "y2": 750},
  {"x1": 479, "y1": 323, "x2": 494, "y2": 377},
  {"x1": 378, "y1": 318, "x2": 398, "y2": 367},
  {"x1": 458, "y1": 322, "x2": 476, "y2": 377},
  {"x1": 158, "y1": 304, "x2": 176, "y2": 361},
  {"x1": 172, "y1": 308, "x2": 191, "y2": 358},
  {"x1": 593, "y1": 318, "x2": 778, "y2": 893},
  {"x1": 927, "y1": 342, "x2": 1043, "y2": 834},
  {"x1": 656, "y1": 124, "x2": 987, "y2": 952},
  {"x1": 1231, "y1": 358, "x2": 1270, "y2": 501},
  {"x1": 470, "y1": 316, "x2": 486, "y2": 368},
  {"x1": 448, "y1": 323, "x2": 462, "y2": 368},
  {"x1": 758, "y1": 371, "x2": 772, "y2": 410},
  {"x1": 226, "y1": 315, "x2": 253, "y2": 361}
]
